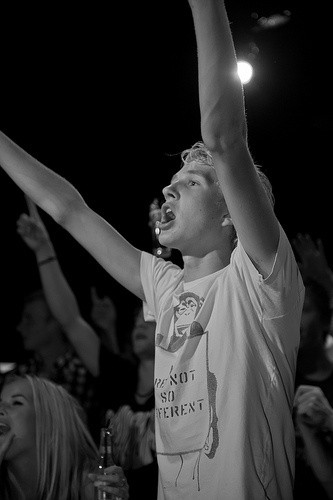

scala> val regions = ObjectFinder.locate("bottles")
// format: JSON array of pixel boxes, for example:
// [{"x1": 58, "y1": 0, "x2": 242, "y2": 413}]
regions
[{"x1": 93, "y1": 427, "x2": 115, "y2": 500}]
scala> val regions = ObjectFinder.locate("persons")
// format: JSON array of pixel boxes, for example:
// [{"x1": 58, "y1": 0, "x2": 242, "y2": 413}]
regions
[
  {"x1": 0, "y1": 0, "x2": 306, "y2": 500},
  {"x1": 291, "y1": 233, "x2": 333, "y2": 500},
  {"x1": 19, "y1": 297, "x2": 99, "y2": 440},
  {"x1": 91, "y1": 284, "x2": 120, "y2": 355},
  {"x1": 16, "y1": 193, "x2": 157, "y2": 500},
  {"x1": 0, "y1": 373, "x2": 130, "y2": 500}
]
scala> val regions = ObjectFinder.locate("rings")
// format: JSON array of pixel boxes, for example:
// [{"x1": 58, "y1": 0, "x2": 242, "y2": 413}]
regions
[{"x1": 122, "y1": 479, "x2": 125, "y2": 486}]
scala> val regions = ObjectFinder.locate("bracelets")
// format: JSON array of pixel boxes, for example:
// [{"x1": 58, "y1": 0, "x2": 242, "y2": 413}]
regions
[{"x1": 38, "y1": 255, "x2": 57, "y2": 266}]
